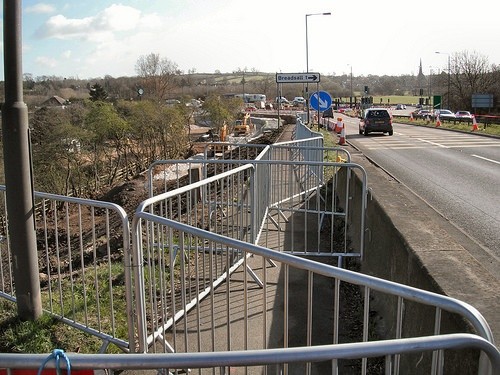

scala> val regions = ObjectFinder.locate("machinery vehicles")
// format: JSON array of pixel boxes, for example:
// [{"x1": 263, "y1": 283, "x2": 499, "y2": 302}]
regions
[{"x1": 233, "y1": 112, "x2": 255, "y2": 136}]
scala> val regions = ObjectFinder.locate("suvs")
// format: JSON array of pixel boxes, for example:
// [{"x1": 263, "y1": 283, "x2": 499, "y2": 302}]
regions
[{"x1": 357, "y1": 108, "x2": 394, "y2": 136}]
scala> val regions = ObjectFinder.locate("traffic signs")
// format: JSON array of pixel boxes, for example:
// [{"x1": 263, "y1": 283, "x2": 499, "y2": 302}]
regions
[{"x1": 275, "y1": 72, "x2": 321, "y2": 84}]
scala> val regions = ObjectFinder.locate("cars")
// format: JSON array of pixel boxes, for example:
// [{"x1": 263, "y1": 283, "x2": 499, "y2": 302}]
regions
[
  {"x1": 292, "y1": 97, "x2": 306, "y2": 106},
  {"x1": 417, "y1": 110, "x2": 432, "y2": 117},
  {"x1": 416, "y1": 103, "x2": 423, "y2": 108},
  {"x1": 397, "y1": 104, "x2": 406, "y2": 111},
  {"x1": 276, "y1": 97, "x2": 289, "y2": 104},
  {"x1": 456, "y1": 110, "x2": 474, "y2": 121},
  {"x1": 433, "y1": 109, "x2": 456, "y2": 121}
]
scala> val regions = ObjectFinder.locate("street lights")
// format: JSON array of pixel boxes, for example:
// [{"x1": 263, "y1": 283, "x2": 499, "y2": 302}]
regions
[
  {"x1": 306, "y1": 11, "x2": 333, "y2": 124},
  {"x1": 434, "y1": 51, "x2": 450, "y2": 112}
]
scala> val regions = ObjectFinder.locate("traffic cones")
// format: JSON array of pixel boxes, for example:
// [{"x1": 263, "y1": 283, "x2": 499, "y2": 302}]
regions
[
  {"x1": 471, "y1": 114, "x2": 481, "y2": 132},
  {"x1": 409, "y1": 112, "x2": 414, "y2": 122},
  {"x1": 338, "y1": 123, "x2": 349, "y2": 146},
  {"x1": 435, "y1": 114, "x2": 443, "y2": 127}
]
